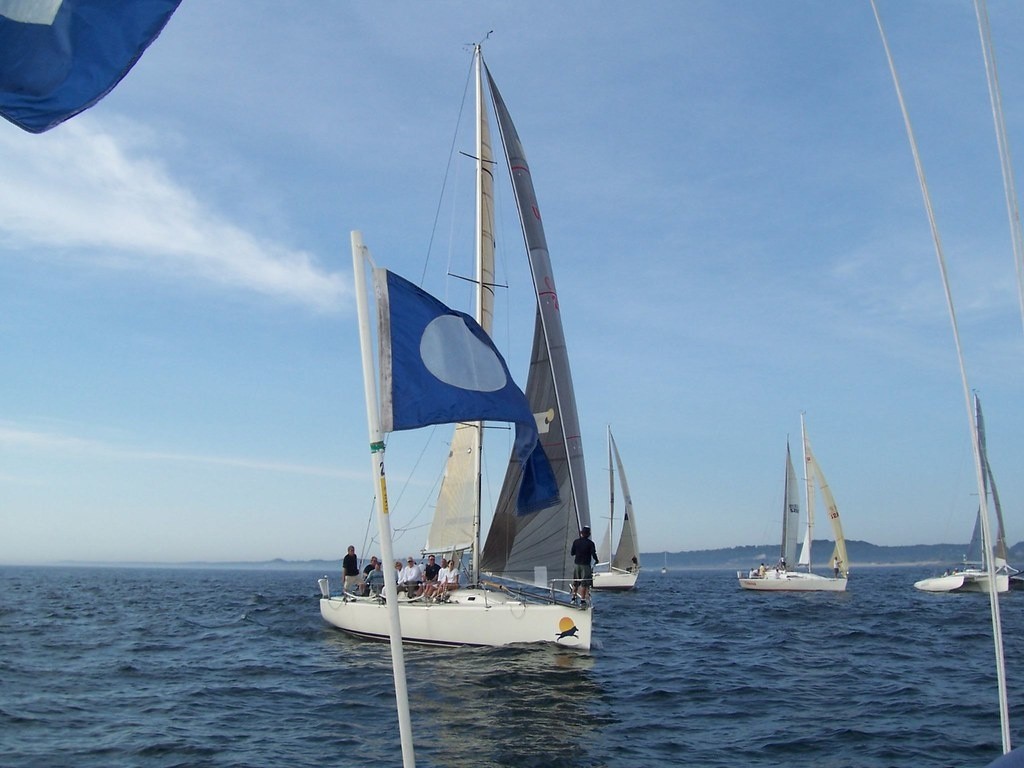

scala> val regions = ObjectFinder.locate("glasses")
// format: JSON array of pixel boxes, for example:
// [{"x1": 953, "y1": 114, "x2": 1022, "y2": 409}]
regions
[
  {"x1": 407, "y1": 561, "x2": 413, "y2": 563},
  {"x1": 430, "y1": 559, "x2": 435, "y2": 561},
  {"x1": 348, "y1": 549, "x2": 354, "y2": 551},
  {"x1": 449, "y1": 563, "x2": 453, "y2": 565}
]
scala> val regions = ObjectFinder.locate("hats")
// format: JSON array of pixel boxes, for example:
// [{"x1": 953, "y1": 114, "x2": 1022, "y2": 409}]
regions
[{"x1": 583, "y1": 525, "x2": 590, "y2": 534}]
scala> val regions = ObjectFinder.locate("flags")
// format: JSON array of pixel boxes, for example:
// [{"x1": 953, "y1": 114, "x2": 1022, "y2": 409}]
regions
[{"x1": 372, "y1": 262, "x2": 566, "y2": 516}]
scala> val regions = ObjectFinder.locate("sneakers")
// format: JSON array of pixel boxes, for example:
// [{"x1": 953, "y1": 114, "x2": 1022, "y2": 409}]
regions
[
  {"x1": 580, "y1": 602, "x2": 588, "y2": 607},
  {"x1": 570, "y1": 600, "x2": 578, "y2": 605}
]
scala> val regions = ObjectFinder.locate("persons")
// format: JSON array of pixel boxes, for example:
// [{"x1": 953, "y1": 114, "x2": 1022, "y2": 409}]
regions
[
  {"x1": 341, "y1": 546, "x2": 460, "y2": 600},
  {"x1": 570, "y1": 526, "x2": 599, "y2": 608},
  {"x1": 944, "y1": 567, "x2": 958, "y2": 577},
  {"x1": 749, "y1": 556, "x2": 843, "y2": 579},
  {"x1": 632, "y1": 555, "x2": 637, "y2": 571}
]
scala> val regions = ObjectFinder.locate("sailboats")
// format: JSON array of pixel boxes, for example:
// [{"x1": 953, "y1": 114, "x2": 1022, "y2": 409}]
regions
[
  {"x1": 913, "y1": 387, "x2": 1023, "y2": 595},
  {"x1": 736, "y1": 410, "x2": 855, "y2": 592},
  {"x1": 318, "y1": 38, "x2": 597, "y2": 651},
  {"x1": 583, "y1": 424, "x2": 641, "y2": 591}
]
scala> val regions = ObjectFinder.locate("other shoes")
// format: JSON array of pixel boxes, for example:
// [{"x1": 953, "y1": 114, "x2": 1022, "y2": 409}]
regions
[{"x1": 343, "y1": 597, "x2": 348, "y2": 603}]
